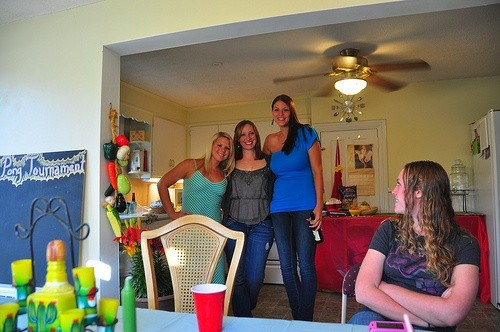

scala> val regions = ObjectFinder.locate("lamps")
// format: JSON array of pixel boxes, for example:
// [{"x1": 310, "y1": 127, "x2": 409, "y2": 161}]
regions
[{"x1": 334, "y1": 70, "x2": 367, "y2": 96}]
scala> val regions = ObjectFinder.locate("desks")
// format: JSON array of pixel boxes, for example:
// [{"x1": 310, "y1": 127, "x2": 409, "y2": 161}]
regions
[
  {"x1": 17, "y1": 305, "x2": 437, "y2": 332},
  {"x1": 314, "y1": 213, "x2": 491, "y2": 305}
]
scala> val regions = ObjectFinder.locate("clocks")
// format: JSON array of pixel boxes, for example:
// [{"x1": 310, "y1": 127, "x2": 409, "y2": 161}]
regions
[{"x1": 331, "y1": 93, "x2": 365, "y2": 122}]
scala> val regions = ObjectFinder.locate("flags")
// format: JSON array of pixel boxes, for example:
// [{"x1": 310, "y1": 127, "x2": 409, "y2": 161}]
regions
[{"x1": 331, "y1": 142, "x2": 343, "y2": 200}]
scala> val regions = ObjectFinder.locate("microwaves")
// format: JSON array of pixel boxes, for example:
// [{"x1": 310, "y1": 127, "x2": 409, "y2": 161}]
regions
[{"x1": 147, "y1": 184, "x2": 183, "y2": 208}]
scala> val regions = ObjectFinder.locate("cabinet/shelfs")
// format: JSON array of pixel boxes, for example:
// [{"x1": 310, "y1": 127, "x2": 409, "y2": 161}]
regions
[
  {"x1": 151, "y1": 116, "x2": 188, "y2": 179},
  {"x1": 119, "y1": 115, "x2": 154, "y2": 179},
  {"x1": 188, "y1": 114, "x2": 311, "y2": 159}
]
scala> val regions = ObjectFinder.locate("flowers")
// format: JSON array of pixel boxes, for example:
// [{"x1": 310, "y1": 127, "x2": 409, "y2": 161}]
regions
[{"x1": 112, "y1": 217, "x2": 173, "y2": 299}]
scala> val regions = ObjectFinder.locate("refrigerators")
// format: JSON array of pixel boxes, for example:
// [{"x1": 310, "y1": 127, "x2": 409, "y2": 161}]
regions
[{"x1": 470, "y1": 108, "x2": 500, "y2": 310}]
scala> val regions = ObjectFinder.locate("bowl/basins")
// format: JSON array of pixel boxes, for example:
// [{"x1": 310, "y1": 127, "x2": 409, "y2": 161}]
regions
[
  {"x1": 349, "y1": 209, "x2": 361, "y2": 217},
  {"x1": 326, "y1": 203, "x2": 341, "y2": 211}
]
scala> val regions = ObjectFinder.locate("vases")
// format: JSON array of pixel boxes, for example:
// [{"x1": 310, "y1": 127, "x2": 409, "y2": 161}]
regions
[{"x1": 134, "y1": 295, "x2": 174, "y2": 311}]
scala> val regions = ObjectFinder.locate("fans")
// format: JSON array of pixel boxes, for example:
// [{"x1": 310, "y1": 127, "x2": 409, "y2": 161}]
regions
[{"x1": 273, "y1": 48, "x2": 431, "y2": 97}]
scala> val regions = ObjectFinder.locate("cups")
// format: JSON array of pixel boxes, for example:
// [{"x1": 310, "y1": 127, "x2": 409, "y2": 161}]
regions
[{"x1": 190, "y1": 283, "x2": 227, "y2": 332}]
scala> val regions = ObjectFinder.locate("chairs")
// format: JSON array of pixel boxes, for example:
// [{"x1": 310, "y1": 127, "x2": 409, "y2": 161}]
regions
[
  {"x1": 141, "y1": 214, "x2": 245, "y2": 317},
  {"x1": 341, "y1": 265, "x2": 361, "y2": 324}
]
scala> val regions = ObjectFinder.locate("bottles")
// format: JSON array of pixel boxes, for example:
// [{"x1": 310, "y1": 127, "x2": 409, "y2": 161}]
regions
[
  {"x1": 310, "y1": 211, "x2": 323, "y2": 243},
  {"x1": 130, "y1": 193, "x2": 136, "y2": 214},
  {"x1": 449, "y1": 159, "x2": 468, "y2": 195}
]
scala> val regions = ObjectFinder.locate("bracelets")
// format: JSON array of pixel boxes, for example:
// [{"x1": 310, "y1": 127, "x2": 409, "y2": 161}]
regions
[{"x1": 427, "y1": 322, "x2": 431, "y2": 328}]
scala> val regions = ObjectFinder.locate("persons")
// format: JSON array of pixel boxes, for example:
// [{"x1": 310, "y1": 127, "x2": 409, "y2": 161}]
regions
[
  {"x1": 157, "y1": 94, "x2": 323, "y2": 321},
  {"x1": 355, "y1": 144, "x2": 372, "y2": 167},
  {"x1": 347, "y1": 161, "x2": 481, "y2": 332}
]
